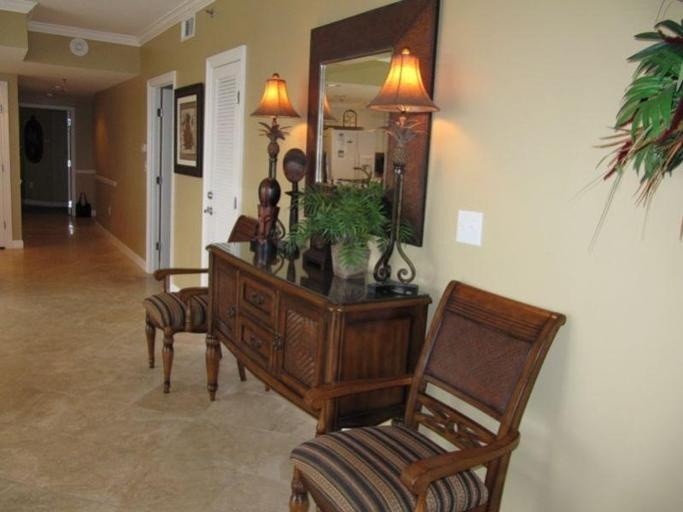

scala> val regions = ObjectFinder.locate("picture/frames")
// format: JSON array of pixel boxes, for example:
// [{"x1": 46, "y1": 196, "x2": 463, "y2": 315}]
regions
[{"x1": 174, "y1": 82, "x2": 204, "y2": 177}]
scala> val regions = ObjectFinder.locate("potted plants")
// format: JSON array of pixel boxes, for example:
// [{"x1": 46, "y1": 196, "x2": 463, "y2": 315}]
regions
[{"x1": 284, "y1": 179, "x2": 418, "y2": 280}]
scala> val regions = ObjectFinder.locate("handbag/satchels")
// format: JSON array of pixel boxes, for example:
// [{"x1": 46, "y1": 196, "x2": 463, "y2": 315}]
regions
[{"x1": 75, "y1": 191, "x2": 91, "y2": 217}]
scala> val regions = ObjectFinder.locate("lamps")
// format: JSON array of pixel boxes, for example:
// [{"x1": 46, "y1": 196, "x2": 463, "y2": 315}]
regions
[
  {"x1": 250, "y1": 73, "x2": 300, "y2": 242},
  {"x1": 365, "y1": 47, "x2": 441, "y2": 285},
  {"x1": 323, "y1": 93, "x2": 337, "y2": 121}
]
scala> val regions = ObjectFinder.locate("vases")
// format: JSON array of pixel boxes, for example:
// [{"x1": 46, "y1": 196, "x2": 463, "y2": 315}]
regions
[{"x1": 327, "y1": 276, "x2": 367, "y2": 305}]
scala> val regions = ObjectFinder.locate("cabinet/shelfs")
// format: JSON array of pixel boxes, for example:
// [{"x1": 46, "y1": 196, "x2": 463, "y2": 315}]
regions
[{"x1": 204, "y1": 241, "x2": 432, "y2": 433}]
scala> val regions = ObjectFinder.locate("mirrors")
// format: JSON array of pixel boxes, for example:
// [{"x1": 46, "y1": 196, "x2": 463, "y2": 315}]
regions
[{"x1": 303, "y1": 0, "x2": 439, "y2": 247}]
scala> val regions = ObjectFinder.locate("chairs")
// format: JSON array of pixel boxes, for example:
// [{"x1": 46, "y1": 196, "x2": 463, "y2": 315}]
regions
[
  {"x1": 141, "y1": 214, "x2": 259, "y2": 393},
  {"x1": 289, "y1": 279, "x2": 567, "y2": 512}
]
